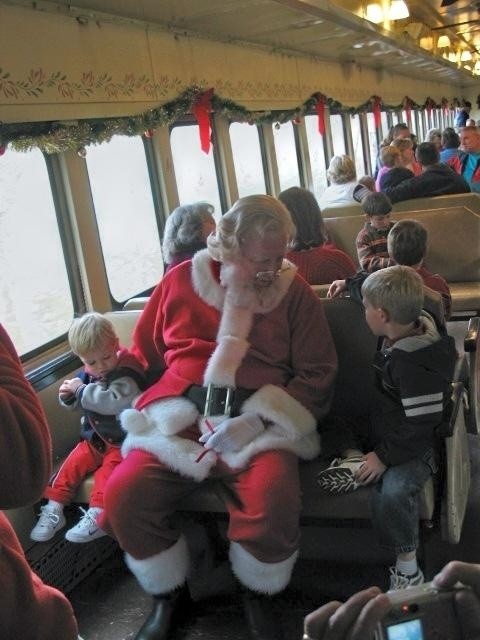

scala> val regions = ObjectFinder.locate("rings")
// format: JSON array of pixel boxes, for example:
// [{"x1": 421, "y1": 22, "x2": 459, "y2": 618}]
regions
[{"x1": 301, "y1": 629, "x2": 314, "y2": 640}]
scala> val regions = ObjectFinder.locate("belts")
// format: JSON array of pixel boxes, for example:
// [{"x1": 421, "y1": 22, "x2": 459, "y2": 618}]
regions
[{"x1": 184, "y1": 384, "x2": 258, "y2": 417}]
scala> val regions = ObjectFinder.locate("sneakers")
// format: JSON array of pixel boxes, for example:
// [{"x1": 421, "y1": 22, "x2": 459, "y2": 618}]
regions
[
  {"x1": 65, "y1": 506, "x2": 110, "y2": 544},
  {"x1": 387, "y1": 565, "x2": 425, "y2": 591},
  {"x1": 316, "y1": 455, "x2": 368, "y2": 496},
  {"x1": 30, "y1": 504, "x2": 67, "y2": 542}
]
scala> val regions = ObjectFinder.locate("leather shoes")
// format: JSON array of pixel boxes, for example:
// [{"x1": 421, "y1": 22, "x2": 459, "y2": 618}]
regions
[
  {"x1": 243, "y1": 590, "x2": 284, "y2": 640},
  {"x1": 134, "y1": 578, "x2": 195, "y2": 640}
]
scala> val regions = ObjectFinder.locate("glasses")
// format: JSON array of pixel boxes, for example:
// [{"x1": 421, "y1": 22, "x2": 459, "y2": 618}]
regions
[{"x1": 242, "y1": 252, "x2": 292, "y2": 284}]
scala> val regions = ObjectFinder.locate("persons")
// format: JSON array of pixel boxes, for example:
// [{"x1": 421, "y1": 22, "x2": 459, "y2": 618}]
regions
[
  {"x1": 297, "y1": 558, "x2": 480, "y2": 640},
  {"x1": 1, "y1": 322, "x2": 88, "y2": 639},
  {"x1": 320, "y1": 101, "x2": 478, "y2": 204},
  {"x1": 28, "y1": 310, "x2": 147, "y2": 544},
  {"x1": 318, "y1": 259, "x2": 460, "y2": 595},
  {"x1": 103, "y1": 194, "x2": 342, "y2": 638},
  {"x1": 278, "y1": 187, "x2": 452, "y2": 319},
  {"x1": 160, "y1": 197, "x2": 219, "y2": 275}
]
